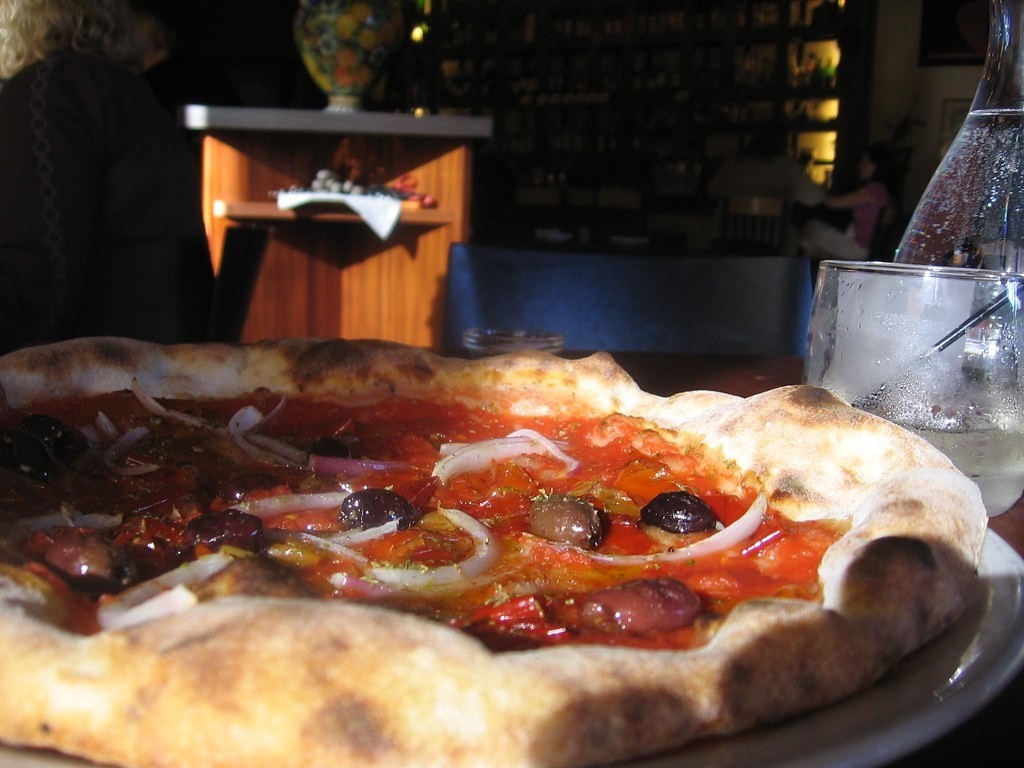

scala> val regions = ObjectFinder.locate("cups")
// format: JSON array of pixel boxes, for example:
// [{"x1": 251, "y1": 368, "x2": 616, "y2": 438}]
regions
[
  {"x1": 461, "y1": 329, "x2": 568, "y2": 364},
  {"x1": 812, "y1": 259, "x2": 1024, "y2": 515}
]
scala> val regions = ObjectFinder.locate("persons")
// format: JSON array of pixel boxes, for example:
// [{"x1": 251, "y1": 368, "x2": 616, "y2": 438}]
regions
[
  {"x1": 798, "y1": 145, "x2": 896, "y2": 261},
  {"x1": 0, "y1": 0, "x2": 213, "y2": 357},
  {"x1": 708, "y1": 123, "x2": 822, "y2": 257}
]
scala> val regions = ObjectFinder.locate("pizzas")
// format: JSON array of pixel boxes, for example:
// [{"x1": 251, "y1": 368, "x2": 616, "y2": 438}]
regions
[{"x1": 1, "y1": 338, "x2": 986, "y2": 768}]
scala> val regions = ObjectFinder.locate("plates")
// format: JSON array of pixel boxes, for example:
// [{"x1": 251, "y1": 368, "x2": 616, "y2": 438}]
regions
[{"x1": 1, "y1": 389, "x2": 1024, "y2": 768}]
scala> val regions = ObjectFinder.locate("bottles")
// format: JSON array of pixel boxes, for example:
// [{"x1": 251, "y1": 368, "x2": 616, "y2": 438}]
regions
[{"x1": 895, "y1": 0, "x2": 1024, "y2": 271}]
